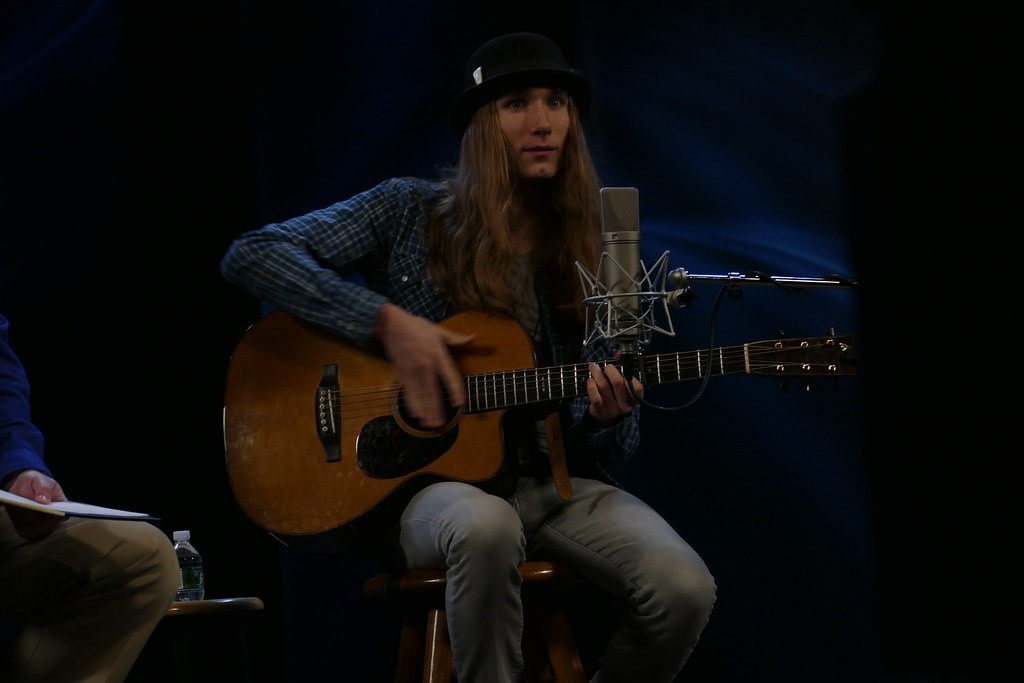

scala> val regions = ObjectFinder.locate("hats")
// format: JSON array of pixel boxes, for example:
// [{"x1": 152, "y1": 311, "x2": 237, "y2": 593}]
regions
[{"x1": 443, "y1": 31, "x2": 592, "y2": 136}]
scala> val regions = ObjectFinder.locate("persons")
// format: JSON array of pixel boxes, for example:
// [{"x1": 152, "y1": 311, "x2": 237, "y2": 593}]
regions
[
  {"x1": 0, "y1": 315, "x2": 181, "y2": 683},
  {"x1": 221, "y1": 32, "x2": 719, "y2": 683}
]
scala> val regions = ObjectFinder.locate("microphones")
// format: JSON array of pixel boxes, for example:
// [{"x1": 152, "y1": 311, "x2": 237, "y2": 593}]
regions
[{"x1": 599, "y1": 187, "x2": 641, "y2": 384}]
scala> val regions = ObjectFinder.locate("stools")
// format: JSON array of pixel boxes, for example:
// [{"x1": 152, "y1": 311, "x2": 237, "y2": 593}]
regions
[
  {"x1": 164, "y1": 596, "x2": 265, "y2": 683},
  {"x1": 393, "y1": 561, "x2": 587, "y2": 683}
]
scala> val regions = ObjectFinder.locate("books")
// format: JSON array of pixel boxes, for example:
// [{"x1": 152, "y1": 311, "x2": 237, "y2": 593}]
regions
[{"x1": 1, "y1": 489, "x2": 162, "y2": 520}]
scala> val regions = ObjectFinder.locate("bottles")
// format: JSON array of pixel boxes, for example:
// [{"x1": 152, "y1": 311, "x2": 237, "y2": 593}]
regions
[{"x1": 173, "y1": 531, "x2": 203, "y2": 603}]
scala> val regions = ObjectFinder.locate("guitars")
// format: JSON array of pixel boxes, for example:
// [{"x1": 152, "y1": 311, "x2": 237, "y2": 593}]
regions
[{"x1": 223, "y1": 305, "x2": 858, "y2": 537}]
